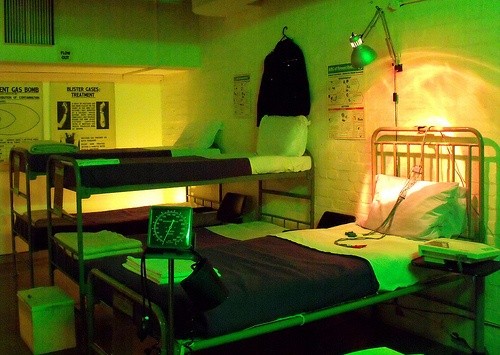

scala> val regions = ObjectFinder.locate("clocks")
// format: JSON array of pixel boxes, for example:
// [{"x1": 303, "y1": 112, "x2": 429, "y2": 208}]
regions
[{"x1": 146, "y1": 204, "x2": 193, "y2": 250}]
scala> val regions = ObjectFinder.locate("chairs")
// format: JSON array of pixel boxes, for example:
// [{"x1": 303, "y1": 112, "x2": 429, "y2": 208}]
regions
[
  {"x1": 201, "y1": 192, "x2": 246, "y2": 224},
  {"x1": 282, "y1": 211, "x2": 356, "y2": 233}
]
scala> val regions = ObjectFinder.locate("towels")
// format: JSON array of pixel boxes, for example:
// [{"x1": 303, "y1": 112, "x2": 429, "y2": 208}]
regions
[
  {"x1": 205, "y1": 221, "x2": 290, "y2": 241},
  {"x1": 18, "y1": 140, "x2": 79, "y2": 154},
  {"x1": 76, "y1": 158, "x2": 120, "y2": 166},
  {"x1": 122, "y1": 256, "x2": 221, "y2": 284},
  {"x1": 54, "y1": 230, "x2": 143, "y2": 259},
  {"x1": 22, "y1": 209, "x2": 74, "y2": 227}
]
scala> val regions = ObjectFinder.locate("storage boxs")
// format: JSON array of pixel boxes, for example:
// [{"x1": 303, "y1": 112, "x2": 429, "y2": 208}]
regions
[{"x1": 16, "y1": 287, "x2": 75, "y2": 354}]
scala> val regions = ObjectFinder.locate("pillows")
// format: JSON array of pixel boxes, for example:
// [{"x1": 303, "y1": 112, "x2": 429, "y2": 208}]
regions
[
  {"x1": 363, "y1": 174, "x2": 460, "y2": 241},
  {"x1": 256, "y1": 115, "x2": 309, "y2": 156},
  {"x1": 174, "y1": 120, "x2": 220, "y2": 147}
]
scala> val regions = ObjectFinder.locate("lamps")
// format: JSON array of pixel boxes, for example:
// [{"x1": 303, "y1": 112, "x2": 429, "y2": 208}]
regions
[{"x1": 350, "y1": 6, "x2": 401, "y2": 73}]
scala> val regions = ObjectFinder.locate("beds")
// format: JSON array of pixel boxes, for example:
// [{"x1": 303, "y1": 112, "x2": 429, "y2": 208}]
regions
[{"x1": 8, "y1": 125, "x2": 487, "y2": 355}]
